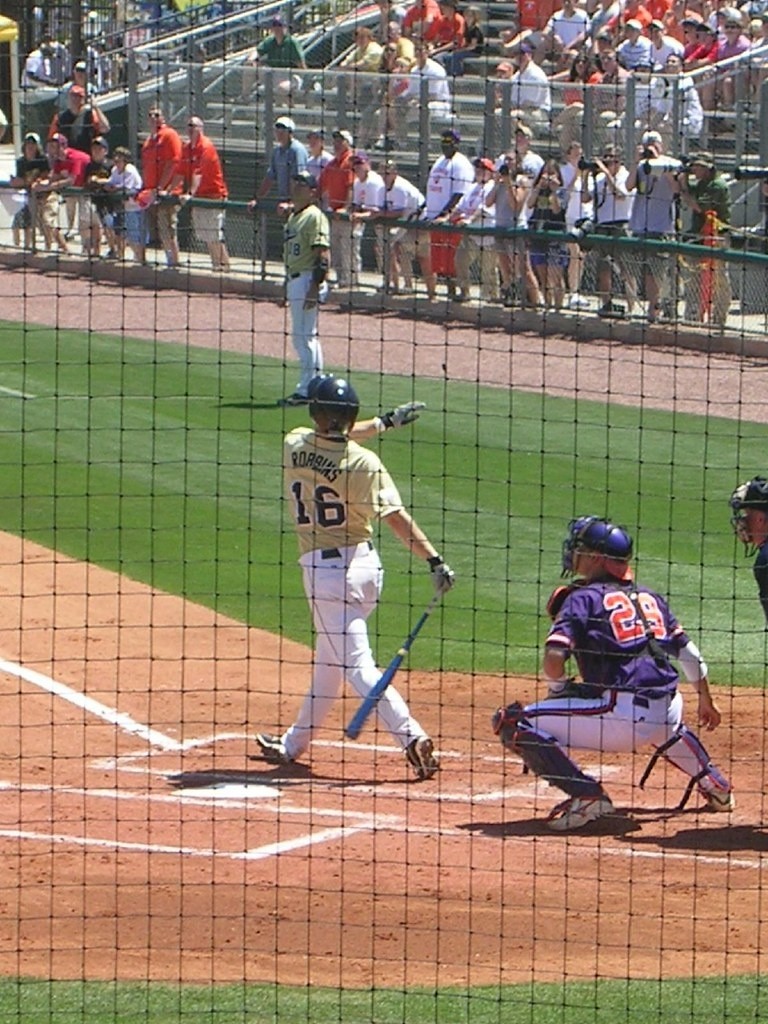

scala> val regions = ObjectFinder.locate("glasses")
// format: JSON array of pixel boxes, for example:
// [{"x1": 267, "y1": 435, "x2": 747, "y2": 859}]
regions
[
  {"x1": 604, "y1": 154, "x2": 614, "y2": 158},
  {"x1": 148, "y1": 113, "x2": 160, "y2": 117}
]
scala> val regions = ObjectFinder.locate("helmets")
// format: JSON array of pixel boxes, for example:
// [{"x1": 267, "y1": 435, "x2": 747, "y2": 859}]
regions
[
  {"x1": 307, "y1": 375, "x2": 359, "y2": 419},
  {"x1": 734, "y1": 478, "x2": 768, "y2": 508},
  {"x1": 579, "y1": 519, "x2": 633, "y2": 582}
]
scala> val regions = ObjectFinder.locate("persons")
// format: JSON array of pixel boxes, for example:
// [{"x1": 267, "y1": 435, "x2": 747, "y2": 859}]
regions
[
  {"x1": 254, "y1": 371, "x2": 456, "y2": 780},
  {"x1": 484, "y1": 151, "x2": 540, "y2": 305},
  {"x1": 558, "y1": 142, "x2": 595, "y2": 306},
  {"x1": 12, "y1": 132, "x2": 149, "y2": 265},
  {"x1": 491, "y1": 516, "x2": 734, "y2": 831},
  {"x1": 527, "y1": 158, "x2": 568, "y2": 309},
  {"x1": 499, "y1": 122, "x2": 545, "y2": 221},
  {"x1": 627, "y1": 128, "x2": 732, "y2": 326},
  {"x1": 241, "y1": 14, "x2": 307, "y2": 106},
  {"x1": 729, "y1": 476, "x2": 768, "y2": 622},
  {"x1": 246, "y1": 117, "x2": 309, "y2": 222},
  {"x1": 302, "y1": 130, "x2": 435, "y2": 295},
  {"x1": 405, "y1": 127, "x2": 474, "y2": 297},
  {"x1": 429, "y1": 157, "x2": 498, "y2": 302},
  {"x1": 46, "y1": 86, "x2": 110, "y2": 240},
  {"x1": 134, "y1": 105, "x2": 229, "y2": 272},
  {"x1": 580, "y1": 144, "x2": 636, "y2": 319},
  {"x1": 20, "y1": 30, "x2": 128, "y2": 110},
  {"x1": 278, "y1": 169, "x2": 331, "y2": 407},
  {"x1": 329, "y1": 0, "x2": 767, "y2": 143}
]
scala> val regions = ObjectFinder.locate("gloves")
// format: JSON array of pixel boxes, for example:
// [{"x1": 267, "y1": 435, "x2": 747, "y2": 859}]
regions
[
  {"x1": 427, "y1": 557, "x2": 456, "y2": 593},
  {"x1": 374, "y1": 401, "x2": 425, "y2": 432}
]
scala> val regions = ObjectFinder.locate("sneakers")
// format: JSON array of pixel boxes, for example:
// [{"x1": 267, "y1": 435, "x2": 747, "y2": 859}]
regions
[
  {"x1": 406, "y1": 737, "x2": 438, "y2": 779},
  {"x1": 545, "y1": 781, "x2": 614, "y2": 830},
  {"x1": 704, "y1": 783, "x2": 736, "y2": 812},
  {"x1": 278, "y1": 393, "x2": 308, "y2": 407},
  {"x1": 256, "y1": 733, "x2": 292, "y2": 764}
]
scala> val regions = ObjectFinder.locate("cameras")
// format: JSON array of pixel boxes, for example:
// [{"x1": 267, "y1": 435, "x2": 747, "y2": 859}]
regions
[
  {"x1": 735, "y1": 166, "x2": 768, "y2": 180},
  {"x1": 577, "y1": 157, "x2": 606, "y2": 172},
  {"x1": 642, "y1": 162, "x2": 693, "y2": 175}
]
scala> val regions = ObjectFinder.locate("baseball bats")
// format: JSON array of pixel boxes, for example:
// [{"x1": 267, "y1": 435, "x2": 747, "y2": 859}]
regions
[{"x1": 341, "y1": 571, "x2": 457, "y2": 739}]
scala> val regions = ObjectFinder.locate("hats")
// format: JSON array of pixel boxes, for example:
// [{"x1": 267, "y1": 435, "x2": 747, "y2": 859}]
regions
[
  {"x1": 275, "y1": 117, "x2": 296, "y2": 132},
  {"x1": 24, "y1": 132, "x2": 41, "y2": 144},
  {"x1": 515, "y1": 127, "x2": 533, "y2": 140},
  {"x1": 291, "y1": 170, "x2": 317, "y2": 188},
  {"x1": 333, "y1": 130, "x2": 353, "y2": 146},
  {"x1": 643, "y1": 132, "x2": 663, "y2": 144},
  {"x1": 92, "y1": 136, "x2": 109, "y2": 153},
  {"x1": 693, "y1": 152, "x2": 714, "y2": 169},
  {"x1": 69, "y1": 86, "x2": 84, "y2": 97},
  {"x1": 442, "y1": 130, "x2": 461, "y2": 142},
  {"x1": 473, "y1": 157, "x2": 495, "y2": 172},
  {"x1": 49, "y1": 133, "x2": 69, "y2": 149}
]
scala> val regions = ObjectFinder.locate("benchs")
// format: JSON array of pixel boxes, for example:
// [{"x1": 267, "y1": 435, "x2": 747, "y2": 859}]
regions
[{"x1": 161, "y1": 1, "x2": 767, "y2": 316}]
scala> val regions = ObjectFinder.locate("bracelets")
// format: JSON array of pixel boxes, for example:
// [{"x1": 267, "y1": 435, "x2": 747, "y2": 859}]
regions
[
  {"x1": 427, "y1": 555, "x2": 442, "y2": 567},
  {"x1": 254, "y1": 193, "x2": 259, "y2": 202},
  {"x1": 189, "y1": 192, "x2": 192, "y2": 195},
  {"x1": 533, "y1": 188, "x2": 536, "y2": 192},
  {"x1": 154, "y1": 186, "x2": 159, "y2": 190}
]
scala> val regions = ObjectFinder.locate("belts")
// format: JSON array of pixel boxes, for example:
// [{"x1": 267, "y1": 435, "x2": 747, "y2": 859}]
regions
[
  {"x1": 285, "y1": 273, "x2": 299, "y2": 282},
  {"x1": 632, "y1": 689, "x2": 676, "y2": 709},
  {"x1": 321, "y1": 542, "x2": 373, "y2": 558}
]
